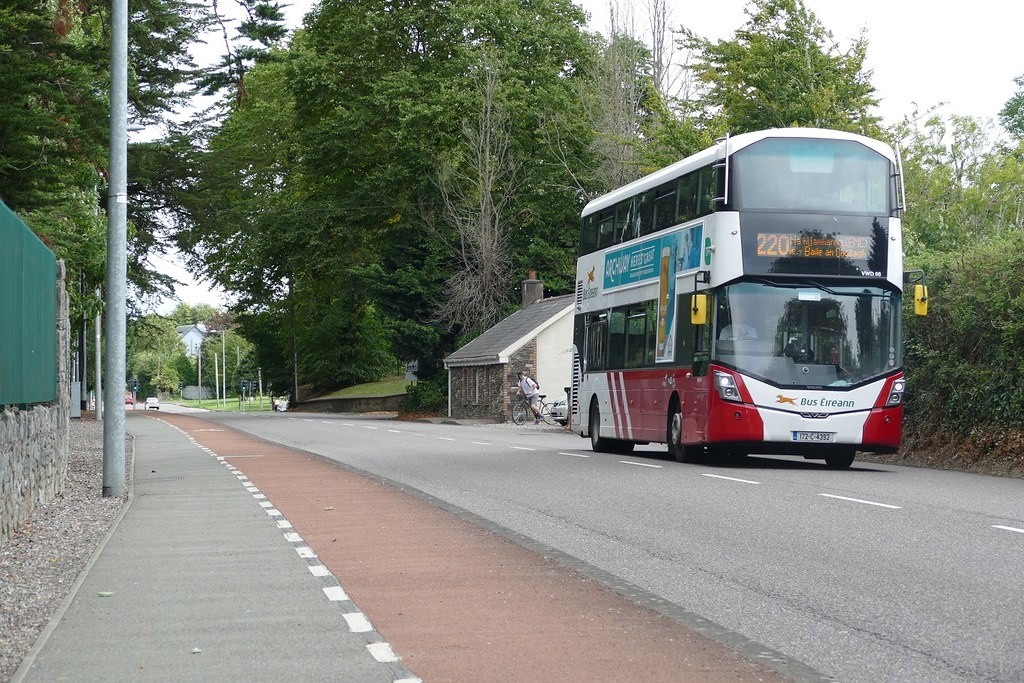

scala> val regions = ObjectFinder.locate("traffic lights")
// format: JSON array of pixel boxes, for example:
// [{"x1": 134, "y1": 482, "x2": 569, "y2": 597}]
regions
[
  {"x1": 251, "y1": 379, "x2": 258, "y2": 392},
  {"x1": 132, "y1": 379, "x2": 137, "y2": 390},
  {"x1": 241, "y1": 380, "x2": 250, "y2": 391}
]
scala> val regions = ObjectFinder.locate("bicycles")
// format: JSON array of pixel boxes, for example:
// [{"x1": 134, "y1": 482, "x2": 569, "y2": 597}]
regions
[{"x1": 511, "y1": 391, "x2": 558, "y2": 426}]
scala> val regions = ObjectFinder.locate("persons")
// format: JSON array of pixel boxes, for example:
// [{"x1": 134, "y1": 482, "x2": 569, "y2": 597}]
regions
[
  {"x1": 516, "y1": 370, "x2": 544, "y2": 425},
  {"x1": 719, "y1": 303, "x2": 758, "y2": 341}
]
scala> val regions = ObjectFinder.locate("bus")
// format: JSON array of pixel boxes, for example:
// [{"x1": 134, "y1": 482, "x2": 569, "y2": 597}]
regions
[{"x1": 568, "y1": 127, "x2": 930, "y2": 467}]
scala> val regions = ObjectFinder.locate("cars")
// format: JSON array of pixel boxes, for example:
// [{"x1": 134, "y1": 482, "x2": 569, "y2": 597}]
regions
[
  {"x1": 145, "y1": 397, "x2": 160, "y2": 410},
  {"x1": 124, "y1": 394, "x2": 135, "y2": 406},
  {"x1": 548, "y1": 395, "x2": 568, "y2": 426}
]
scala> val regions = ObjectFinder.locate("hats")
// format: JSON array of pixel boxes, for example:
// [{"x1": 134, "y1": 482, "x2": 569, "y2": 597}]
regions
[{"x1": 518, "y1": 370, "x2": 526, "y2": 375}]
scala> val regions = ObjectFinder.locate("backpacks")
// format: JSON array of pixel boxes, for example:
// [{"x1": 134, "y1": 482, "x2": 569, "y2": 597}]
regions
[{"x1": 520, "y1": 376, "x2": 539, "y2": 390}]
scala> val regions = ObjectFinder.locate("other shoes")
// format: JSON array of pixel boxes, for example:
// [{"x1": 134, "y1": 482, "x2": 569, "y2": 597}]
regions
[{"x1": 536, "y1": 415, "x2": 544, "y2": 421}]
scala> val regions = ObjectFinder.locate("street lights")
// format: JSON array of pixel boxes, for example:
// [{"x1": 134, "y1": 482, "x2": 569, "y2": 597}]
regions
[{"x1": 209, "y1": 330, "x2": 227, "y2": 408}]
